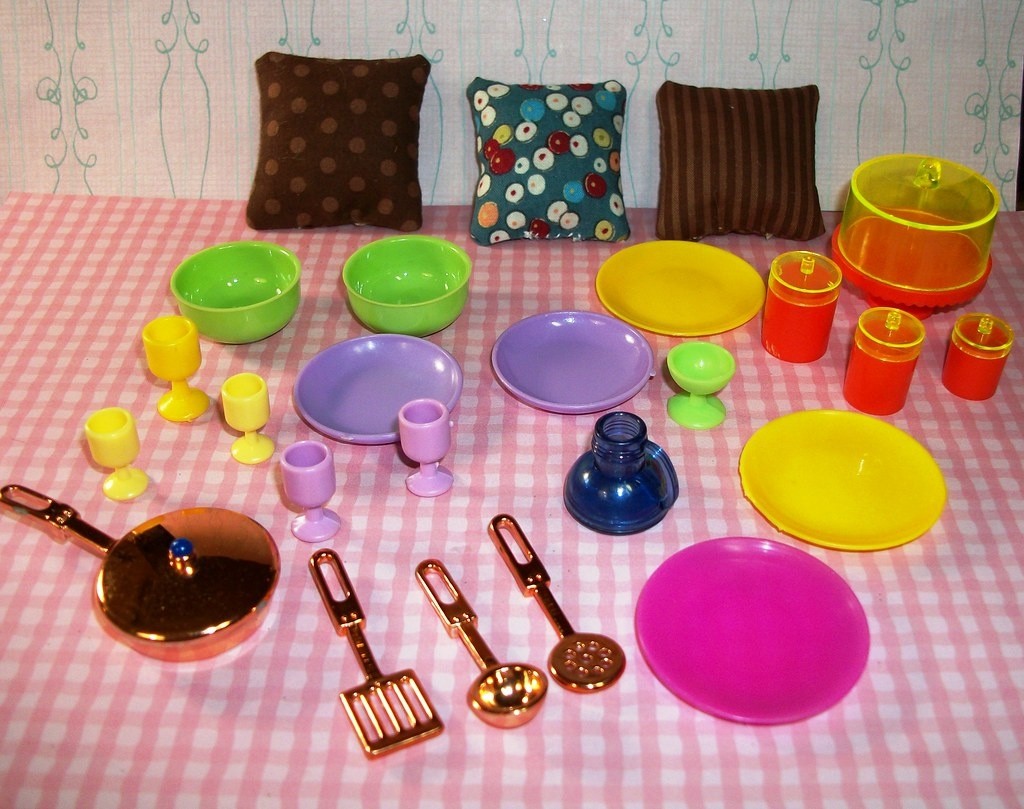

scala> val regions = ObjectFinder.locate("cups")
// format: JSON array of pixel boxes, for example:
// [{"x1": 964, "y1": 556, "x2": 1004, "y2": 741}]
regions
[
  {"x1": 760, "y1": 249, "x2": 842, "y2": 364},
  {"x1": 942, "y1": 313, "x2": 1015, "y2": 401},
  {"x1": 842, "y1": 306, "x2": 926, "y2": 416}
]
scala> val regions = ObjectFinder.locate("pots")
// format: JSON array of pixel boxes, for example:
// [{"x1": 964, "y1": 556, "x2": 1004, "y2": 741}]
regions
[{"x1": 0, "y1": 483, "x2": 283, "y2": 663}]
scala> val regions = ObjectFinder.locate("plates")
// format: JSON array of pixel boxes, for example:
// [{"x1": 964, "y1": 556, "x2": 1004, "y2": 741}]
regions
[
  {"x1": 635, "y1": 536, "x2": 870, "y2": 730},
  {"x1": 490, "y1": 310, "x2": 655, "y2": 416},
  {"x1": 737, "y1": 408, "x2": 948, "y2": 552},
  {"x1": 292, "y1": 332, "x2": 463, "y2": 446},
  {"x1": 594, "y1": 239, "x2": 768, "y2": 338}
]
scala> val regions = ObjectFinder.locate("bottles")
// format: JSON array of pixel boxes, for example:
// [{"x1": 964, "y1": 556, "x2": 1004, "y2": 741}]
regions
[{"x1": 562, "y1": 410, "x2": 679, "y2": 537}]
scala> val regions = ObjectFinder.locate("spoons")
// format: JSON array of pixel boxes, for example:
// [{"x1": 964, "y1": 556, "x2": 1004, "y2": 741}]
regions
[
  {"x1": 488, "y1": 513, "x2": 625, "y2": 690},
  {"x1": 417, "y1": 558, "x2": 548, "y2": 728}
]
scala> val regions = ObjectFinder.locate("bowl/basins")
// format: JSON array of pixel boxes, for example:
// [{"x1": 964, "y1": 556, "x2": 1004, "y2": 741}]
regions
[
  {"x1": 169, "y1": 241, "x2": 302, "y2": 345},
  {"x1": 341, "y1": 234, "x2": 472, "y2": 339}
]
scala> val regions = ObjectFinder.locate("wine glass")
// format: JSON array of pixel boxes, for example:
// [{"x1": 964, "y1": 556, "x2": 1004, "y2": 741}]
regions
[
  {"x1": 398, "y1": 399, "x2": 454, "y2": 498},
  {"x1": 666, "y1": 341, "x2": 736, "y2": 431},
  {"x1": 279, "y1": 440, "x2": 341, "y2": 543},
  {"x1": 221, "y1": 373, "x2": 275, "y2": 466},
  {"x1": 141, "y1": 314, "x2": 211, "y2": 424},
  {"x1": 84, "y1": 407, "x2": 148, "y2": 502}
]
